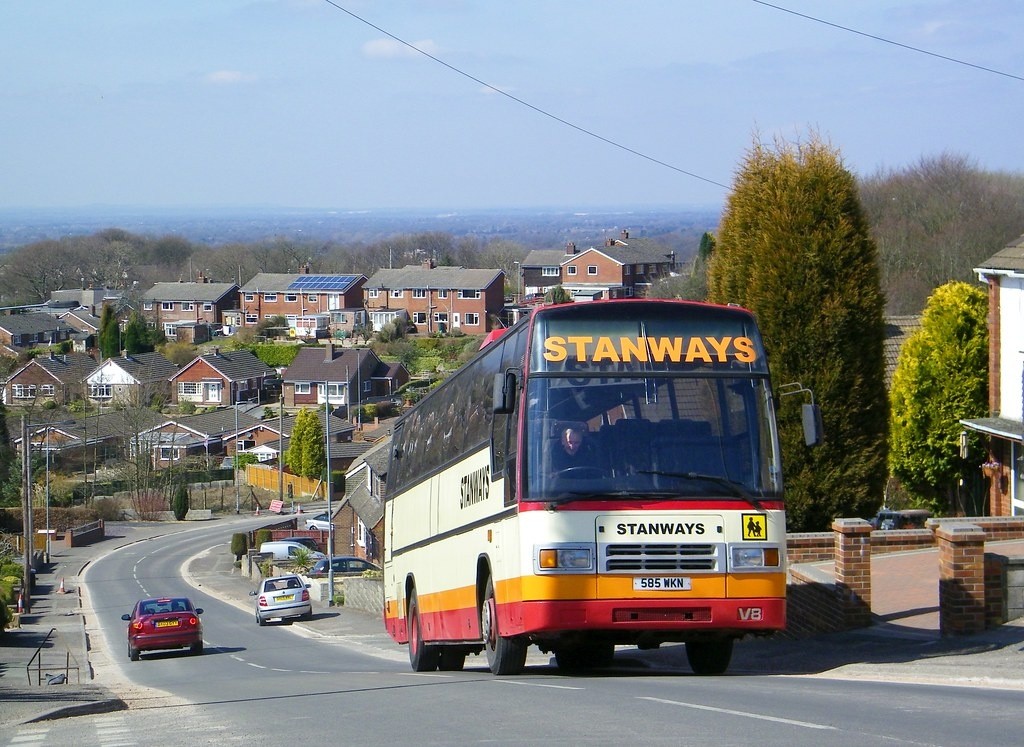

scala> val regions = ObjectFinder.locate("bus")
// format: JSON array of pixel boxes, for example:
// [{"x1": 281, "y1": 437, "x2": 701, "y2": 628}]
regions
[{"x1": 380, "y1": 295, "x2": 823, "y2": 679}]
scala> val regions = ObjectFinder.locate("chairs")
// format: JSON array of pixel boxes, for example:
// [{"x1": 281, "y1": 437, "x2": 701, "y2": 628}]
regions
[
  {"x1": 589, "y1": 418, "x2": 715, "y2": 474},
  {"x1": 544, "y1": 420, "x2": 589, "y2": 473},
  {"x1": 526, "y1": 410, "x2": 550, "y2": 435},
  {"x1": 145, "y1": 609, "x2": 155, "y2": 614},
  {"x1": 287, "y1": 580, "x2": 297, "y2": 589},
  {"x1": 266, "y1": 584, "x2": 276, "y2": 591},
  {"x1": 533, "y1": 416, "x2": 556, "y2": 455},
  {"x1": 174, "y1": 606, "x2": 184, "y2": 612}
]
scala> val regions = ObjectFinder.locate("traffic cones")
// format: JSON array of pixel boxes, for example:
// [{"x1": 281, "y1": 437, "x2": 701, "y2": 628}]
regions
[
  {"x1": 254, "y1": 503, "x2": 261, "y2": 516},
  {"x1": 296, "y1": 503, "x2": 303, "y2": 515},
  {"x1": 18, "y1": 593, "x2": 24, "y2": 613},
  {"x1": 57, "y1": 577, "x2": 66, "y2": 593}
]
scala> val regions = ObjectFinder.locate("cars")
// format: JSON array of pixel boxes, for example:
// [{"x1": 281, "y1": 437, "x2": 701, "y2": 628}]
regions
[
  {"x1": 248, "y1": 575, "x2": 313, "y2": 626},
  {"x1": 307, "y1": 556, "x2": 382, "y2": 579},
  {"x1": 305, "y1": 513, "x2": 332, "y2": 530},
  {"x1": 278, "y1": 537, "x2": 319, "y2": 551},
  {"x1": 121, "y1": 595, "x2": 204, "y2": 660},
  {"x1": 871, "y1": 508, "x2": 933, "y2": 532}
]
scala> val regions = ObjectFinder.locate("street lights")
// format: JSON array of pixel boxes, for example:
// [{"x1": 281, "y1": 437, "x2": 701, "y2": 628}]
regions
[
  {"x1": 235, "y1": 400, "x2": 246, "y2": 514},
  {"x1": 43, "y1": 425, "x2": 86, "y2": 565},
  {"x1": 313, "y1": 380, "x2": 337, "y2": 606},
  {"x1": 17, "y1": 414, "x2": 78, "y2": 615}
]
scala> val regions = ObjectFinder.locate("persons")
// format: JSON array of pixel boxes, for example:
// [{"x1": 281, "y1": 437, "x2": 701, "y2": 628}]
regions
[
  {"x1": 546, "y1": 426, "x2": 602, "y2": 493},
  {"x1": 171, "y1": 601, "x2": 184, "y2": 612}
]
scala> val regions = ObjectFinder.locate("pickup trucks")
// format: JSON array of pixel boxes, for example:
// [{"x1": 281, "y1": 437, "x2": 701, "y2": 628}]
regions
[{"x1": 259, "y1": 540, "x2": 327, "y2": 563}]
